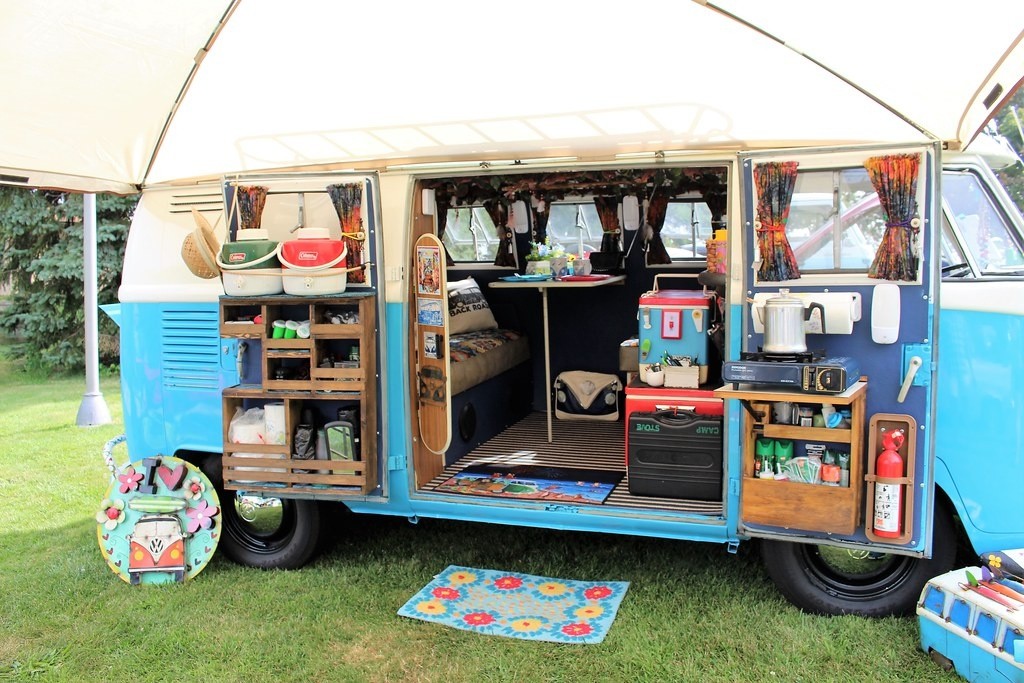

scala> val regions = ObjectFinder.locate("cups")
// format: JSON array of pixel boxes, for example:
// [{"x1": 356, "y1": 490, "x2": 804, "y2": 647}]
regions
[{"x1": 646, "y1": 370, "x2": 664, "y2": 387}]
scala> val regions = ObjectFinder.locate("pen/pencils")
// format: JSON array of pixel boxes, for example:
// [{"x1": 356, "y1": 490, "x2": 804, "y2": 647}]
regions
[{"x1": 660, "y1": 349, "x2": 682, "y2": 367}]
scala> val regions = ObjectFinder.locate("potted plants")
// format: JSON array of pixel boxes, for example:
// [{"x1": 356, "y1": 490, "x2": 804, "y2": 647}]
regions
[
  {"x1": 525, "y1": 242, "x2": 550, "y2": 275},
  {"x1": 547, "y1": 250, "x2": 567, "y2": 276}
]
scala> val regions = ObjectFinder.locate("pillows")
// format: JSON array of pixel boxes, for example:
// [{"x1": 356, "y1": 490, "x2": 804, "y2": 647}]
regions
[{"x1": 446, "y1": 276, "x2": 498, "y2": 335}]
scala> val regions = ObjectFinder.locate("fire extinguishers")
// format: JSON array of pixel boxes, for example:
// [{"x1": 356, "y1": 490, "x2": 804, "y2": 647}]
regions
[{"x1": 873, "y1": 429, "x2": 903, "y2": 538}]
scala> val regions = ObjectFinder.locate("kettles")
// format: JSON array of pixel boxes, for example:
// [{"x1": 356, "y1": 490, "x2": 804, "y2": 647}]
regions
[{"x1": 756, "y1": 288, "x2": 826, "y2": 355}]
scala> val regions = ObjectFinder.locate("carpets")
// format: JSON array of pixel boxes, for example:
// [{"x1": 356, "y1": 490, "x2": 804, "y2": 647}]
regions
[
  {"x1": 433, "y1": 460, "x2": 625, "y2": 504},
  {"x1": 396, "y1": 565, "x2": 630, "y2": 644}
]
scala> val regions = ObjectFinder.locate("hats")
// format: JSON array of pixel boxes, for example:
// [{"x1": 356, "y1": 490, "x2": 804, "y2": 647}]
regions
[{"x1": 182, "y1": 208, "x2": 222, "y2": 279}]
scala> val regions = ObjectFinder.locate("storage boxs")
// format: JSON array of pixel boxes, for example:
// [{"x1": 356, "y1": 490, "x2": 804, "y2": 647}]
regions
[
  {"x1": 637, "y1": 273, "x2": 717, "y2": 388},
  {"x1": 917, "y1": 566, "x2": 1024, "y2": 683}
]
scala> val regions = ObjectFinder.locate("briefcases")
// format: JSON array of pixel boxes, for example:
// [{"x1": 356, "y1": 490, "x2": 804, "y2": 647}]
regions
[{"x1": 628, "y1": 408, "x2": 724, "y2": 502}]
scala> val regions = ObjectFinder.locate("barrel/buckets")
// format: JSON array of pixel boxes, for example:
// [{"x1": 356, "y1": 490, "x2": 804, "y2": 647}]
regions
[
  {"x1": 277, "y1": 227, "x2": 348, "y2": 296},
  {"x1": 214, "y1": 228, "x2": 284, "y2": 297}
]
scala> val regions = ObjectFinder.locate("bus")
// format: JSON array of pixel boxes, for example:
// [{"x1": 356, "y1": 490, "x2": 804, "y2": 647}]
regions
[
  {"x1": 97, "y1": 140, "x2": 1024, "y2": 620},
  {"x1": 543, "y1": 193, "x2": 875, "y2": 272}
]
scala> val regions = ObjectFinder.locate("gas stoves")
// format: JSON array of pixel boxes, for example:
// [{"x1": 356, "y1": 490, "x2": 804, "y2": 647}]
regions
[{"x1": 721, "y1": 345, "x2": 861, "y2": 394}]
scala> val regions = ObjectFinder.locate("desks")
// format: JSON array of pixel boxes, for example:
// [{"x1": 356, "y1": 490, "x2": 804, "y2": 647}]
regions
[{"x1": 489, "y1": 274, "x2": 626, "y2": 443}]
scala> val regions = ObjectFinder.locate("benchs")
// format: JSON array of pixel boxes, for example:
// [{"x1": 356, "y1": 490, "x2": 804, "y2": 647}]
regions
[{"x1": 445, "y1": 329, "x2": 532, "y2": 468}]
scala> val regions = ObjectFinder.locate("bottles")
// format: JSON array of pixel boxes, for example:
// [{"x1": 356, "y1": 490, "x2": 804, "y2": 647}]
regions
[
  {"x1": 774, "y1": 441, "x2": 793, "y2": 474},
  {"x1": 755, "y1": 440, "x2": 774, "y2": 477}
]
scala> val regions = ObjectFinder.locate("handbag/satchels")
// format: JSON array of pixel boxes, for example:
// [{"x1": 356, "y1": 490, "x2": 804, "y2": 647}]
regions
[{"x1": 555, "y1": 370, "x2": 622, "y2": 421}]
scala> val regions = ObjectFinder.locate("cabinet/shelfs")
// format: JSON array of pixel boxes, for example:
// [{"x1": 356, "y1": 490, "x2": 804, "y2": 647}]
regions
[
  {"x1": 219, "y1": 292, "x2": 377, "y2": 496},
  {"x1": 713, "y1": 383, "x2": 866, "y2": 535}
]
